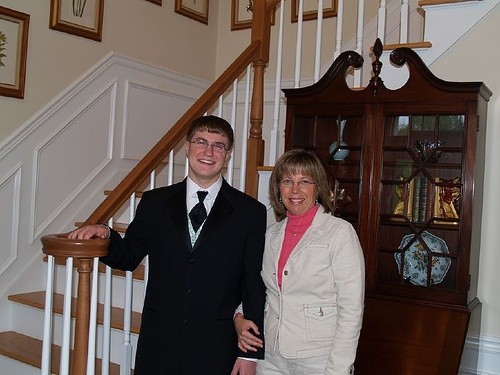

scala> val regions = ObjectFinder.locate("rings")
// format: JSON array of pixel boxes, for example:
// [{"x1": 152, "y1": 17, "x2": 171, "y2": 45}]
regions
[{"x1": 245, "y1": 338, "x2": 248, "y2": 342}]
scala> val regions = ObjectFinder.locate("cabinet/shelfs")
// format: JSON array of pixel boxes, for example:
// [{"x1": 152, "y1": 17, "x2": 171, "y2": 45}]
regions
[{"x1": 282, "y1": 38, "x2": 493, "y2": 375}]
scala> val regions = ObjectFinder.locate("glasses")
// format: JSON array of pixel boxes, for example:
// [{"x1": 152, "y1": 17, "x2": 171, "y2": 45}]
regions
[
  {"x1": 188, "y1": 139, "x2": 229, "y2": 154},
  {"x1": 281, "y1": 180, "x2": 316, "y2": 188}
]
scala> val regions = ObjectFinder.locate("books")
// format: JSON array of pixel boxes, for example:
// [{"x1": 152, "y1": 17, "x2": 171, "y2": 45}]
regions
[{"x1": 413, "y1": 177, "x2": 434, "y2": 224}]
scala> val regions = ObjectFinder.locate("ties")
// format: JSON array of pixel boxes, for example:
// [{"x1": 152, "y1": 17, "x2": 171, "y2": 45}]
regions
[{"x1": 189, "y1": 190, "x2": 209, "y2": 232}]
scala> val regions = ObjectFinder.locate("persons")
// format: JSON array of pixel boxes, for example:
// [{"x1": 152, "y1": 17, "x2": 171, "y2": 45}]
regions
[
  {"x1": 234, "y1": 150, "x2": 366, "y2": 375},
  {"x1": 67, "y1": 114, "x2": 267, "y2": 375}
]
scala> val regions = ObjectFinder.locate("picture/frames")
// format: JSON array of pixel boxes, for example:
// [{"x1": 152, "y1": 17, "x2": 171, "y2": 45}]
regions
[
  {"x1": 175, "y1": 0, "x2": 209, "y2": 25},
  {"x1": 48, "y1": 0, "x2": 104, "y2": 42},
  {"x1": 0, "y1": 6, "x2": 30, "y2": 99},
  {"x1": 291, "y1": 0, "x2": 338, "y2": 23},
  {"x1": 231, "y1": 0, "x2": 276, "y2": 31}
]
